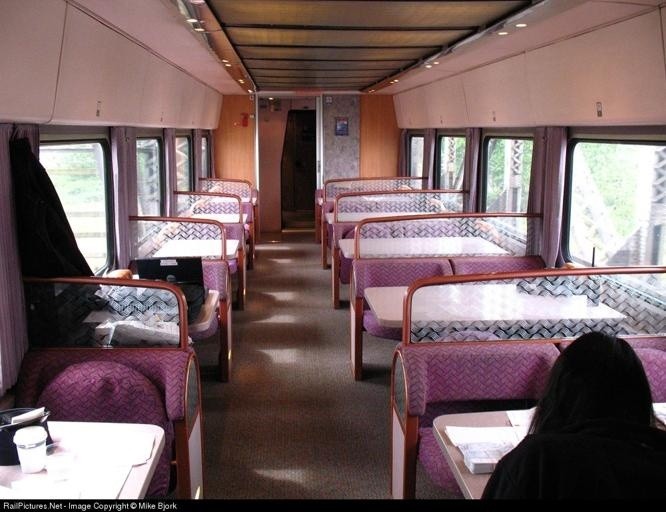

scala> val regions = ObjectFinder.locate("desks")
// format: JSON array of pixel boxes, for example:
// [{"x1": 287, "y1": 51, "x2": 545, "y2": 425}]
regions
[{"x1": 0, "y1": 421, "x2": 167, "y2": 500}]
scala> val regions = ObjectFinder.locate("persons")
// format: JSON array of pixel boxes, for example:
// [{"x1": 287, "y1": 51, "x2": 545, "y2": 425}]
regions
[{"x1": 482, "y1": 332, "x2": 666, "y2": 500}]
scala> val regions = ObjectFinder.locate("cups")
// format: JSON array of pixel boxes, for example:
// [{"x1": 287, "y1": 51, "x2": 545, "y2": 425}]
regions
[{"x1": 13, "y1": 425, "x2": 49, "y2": 476}]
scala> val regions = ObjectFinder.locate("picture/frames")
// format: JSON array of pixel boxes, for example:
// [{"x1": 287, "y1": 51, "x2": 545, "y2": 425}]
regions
[{"x1": 335, "y1": 116, "x2": 349, "y2": 136}]
